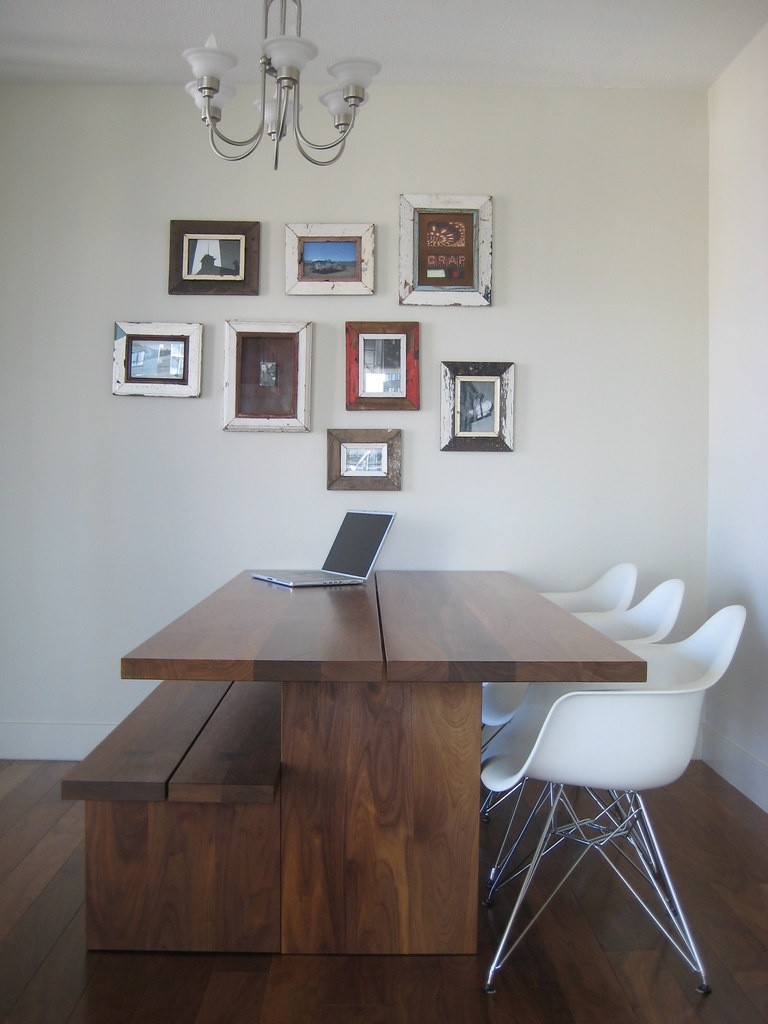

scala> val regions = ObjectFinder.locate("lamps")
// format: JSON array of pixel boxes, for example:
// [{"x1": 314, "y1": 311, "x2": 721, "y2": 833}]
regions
[{"x1": 181, "y1": 0, "x2": 381, "y2": 169}]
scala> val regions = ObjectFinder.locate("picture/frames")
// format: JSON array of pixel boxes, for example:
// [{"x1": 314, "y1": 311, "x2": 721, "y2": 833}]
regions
[
  {"x1": 222, "y1": 319, "x2": 311, "y2": 434},
  {"x1": 111, "y1": 321, "x2": 202, "y2": 398},
  {"x1": 440, "y1": 361, "x2": 514, "y2": 452},
  {"x1": 344, "y1": 321, "x2": 420, "y2": 412},
  {"x1": 397, "y1": 194, "x2": 493, "y2": 307},
  {"x1": 284, "y1": 223, "x2": 375, "y2": 296},
  {"x1": 167, "y1": 219, "x2": 260, "y2": 296},
  {"x1": 326, "y1": 429, "x2": 402, "y2": 491}
]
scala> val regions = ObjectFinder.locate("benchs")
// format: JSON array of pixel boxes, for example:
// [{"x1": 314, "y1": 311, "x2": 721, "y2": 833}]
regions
[{"x1": 60, "y1": 680, "x2": 280, "y2": 952}]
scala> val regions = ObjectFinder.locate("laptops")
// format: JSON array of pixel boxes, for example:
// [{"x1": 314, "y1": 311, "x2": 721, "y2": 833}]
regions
[{"x1": 252, "y1": 510, "x2": 397, "y2": 586}]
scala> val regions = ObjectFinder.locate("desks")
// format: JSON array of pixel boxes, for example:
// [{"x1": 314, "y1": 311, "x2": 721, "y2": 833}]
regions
[{"x1": 120, "y1": 569, "x2": 646, "y2": 954}]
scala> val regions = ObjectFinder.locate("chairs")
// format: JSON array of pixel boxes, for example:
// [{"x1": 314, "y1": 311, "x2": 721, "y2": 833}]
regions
[
  {"x1": 481, "y1": 604, "x2": 745, "y2": 993},
  {"x1": 480, "y1": 578, "x2": 684, "y2": 915},
  {"x1": 478, "y1": 563, "x2": 636, "y2": 823}
]
